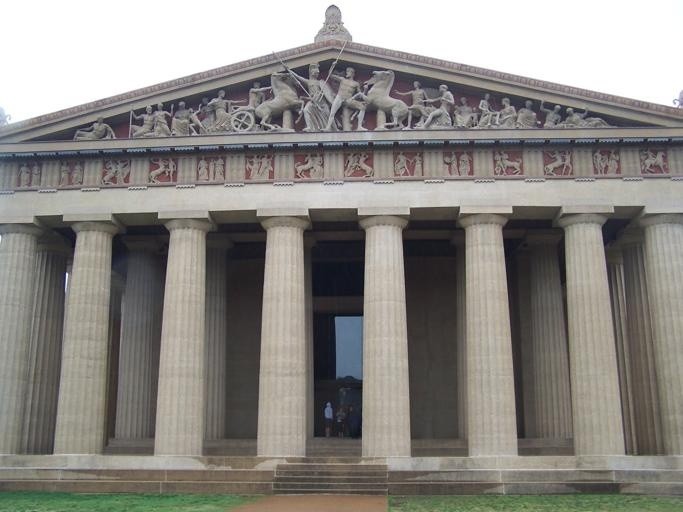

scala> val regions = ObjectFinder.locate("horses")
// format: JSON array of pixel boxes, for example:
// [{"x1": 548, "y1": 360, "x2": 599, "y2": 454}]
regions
[
  {"x1": 346, "y1": 69, "x2": 429, "y2": 130},
  {"x1": 230, "y1": 70, "x2": 306, "y2": 132}
]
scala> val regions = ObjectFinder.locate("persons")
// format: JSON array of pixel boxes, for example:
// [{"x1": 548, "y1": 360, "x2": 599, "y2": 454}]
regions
[
  {"x1": 75, "y1": 117, "x2": 116, "y2": 140},
  {"x1": 324, "y1": 401, "x2": 354, "y2": 439},
  {"x1": 395, "y1": 80, "x2": 609, "y2": 129},
  {"x1": 284, "y1": 62, "x2": 366, "y2": 130},
  {"x1": 131, "y1": 81, "x2": 272, "y2": 137}
]
